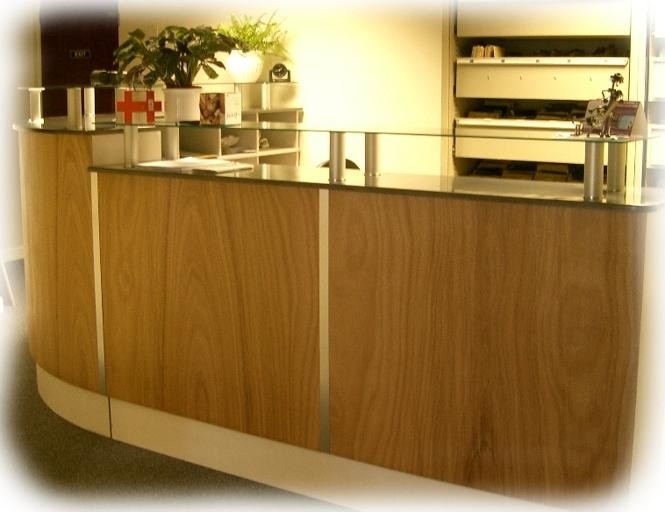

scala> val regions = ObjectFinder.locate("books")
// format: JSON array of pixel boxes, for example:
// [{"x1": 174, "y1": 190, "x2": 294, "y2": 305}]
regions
[
  {"x1": 469, "y1": 161, "x2": 587, "y2": 185},
  {"x1": 467, "y1": 99, "x2": 589, "y2": 125},
  {"x1": 134, "y1": 157, "x2": 254, "y2": 174},
  {"x1": 469, "y1": 39, "x2": 629, "y2": 61}
]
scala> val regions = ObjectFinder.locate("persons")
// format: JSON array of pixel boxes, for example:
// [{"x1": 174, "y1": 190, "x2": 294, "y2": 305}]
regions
[{"x1": 200, "y1": 93, "x2": 225, "y2": 126}]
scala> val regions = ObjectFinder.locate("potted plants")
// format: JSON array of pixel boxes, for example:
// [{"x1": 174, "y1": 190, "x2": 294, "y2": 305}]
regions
[
  {"x1": 113, "y1": 23, "x2": 239, "y2": 122},
  {"x1": 218, "y1": 10, "x2": 290, "y2": 82}
]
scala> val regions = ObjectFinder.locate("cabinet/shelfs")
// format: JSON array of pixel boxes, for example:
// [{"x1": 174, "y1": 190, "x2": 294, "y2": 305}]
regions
[{"x1": 164, "y1": 108, "x2": 304, "y2": 166}]
